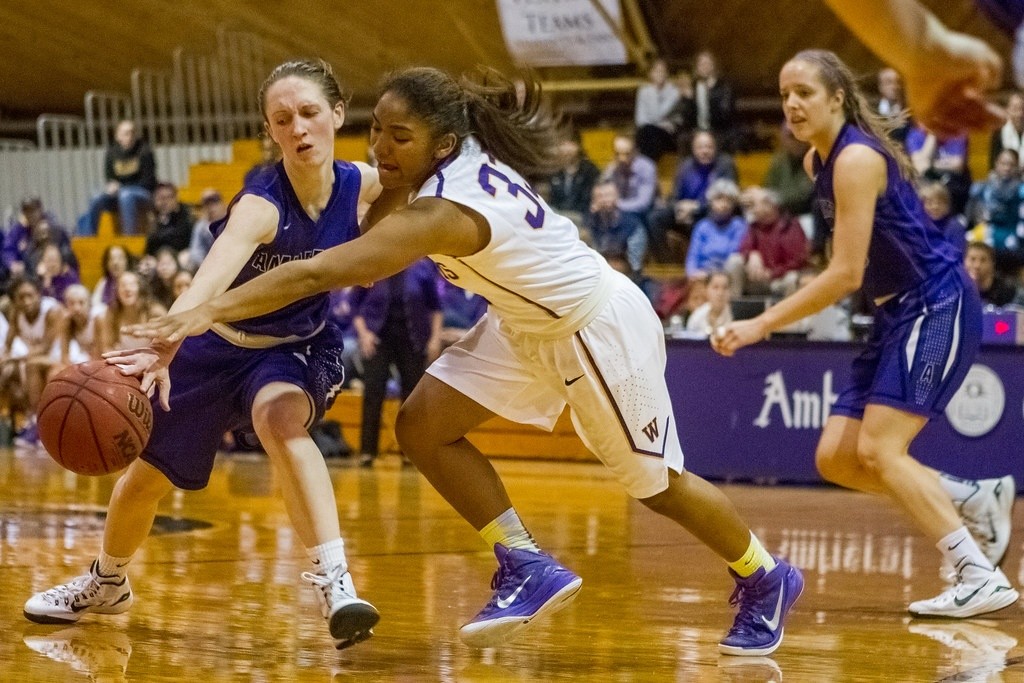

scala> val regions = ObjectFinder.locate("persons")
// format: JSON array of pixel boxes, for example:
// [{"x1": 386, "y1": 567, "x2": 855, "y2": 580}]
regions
[
  {"x1": 711, "y1": 47, "x2": 1018, "y2": 617},
  {"x1": 0, "y1": 0, "x2": 1024, "y2": 683}
]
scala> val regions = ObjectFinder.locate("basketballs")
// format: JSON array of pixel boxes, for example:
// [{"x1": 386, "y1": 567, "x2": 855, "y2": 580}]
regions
[{"x1": 34, "y1": 357, "x2": 155, "y2": 479}]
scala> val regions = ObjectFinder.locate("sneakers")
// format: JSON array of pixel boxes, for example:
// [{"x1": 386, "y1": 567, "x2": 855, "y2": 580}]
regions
[
  {"x1": 22, "y1": 627, "x2": 132, "y2": 683},
  {"x1": 908, "y1": 619, "x2": 1018, "y2": 683},
  {"x1": 300, "y1": 560, "x2": 381, "y2": 651},
  {"x1": 909, "y1": 562, "x2": 1019, "y2": 618},
  {"x1": 718, "y1": 555, "x2": 804, "y2": 656},
  {"x1": 24, "y1": 557, "x2": 133, "y2": 625},
  {"x1": 718, "y1": 654, "x2": 783, "y2": 683},
  {"x1": 459, "y1": 543, "x2": 582, "y2": 650},
  {"x1": 961, "y1": 474, "x2": 1015, "y2": 566}
]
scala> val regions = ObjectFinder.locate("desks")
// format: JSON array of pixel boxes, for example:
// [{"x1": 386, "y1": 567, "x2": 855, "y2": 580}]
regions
[{"x1": 665, "y1": 335, "x2": 1024, "y2": 498}]
